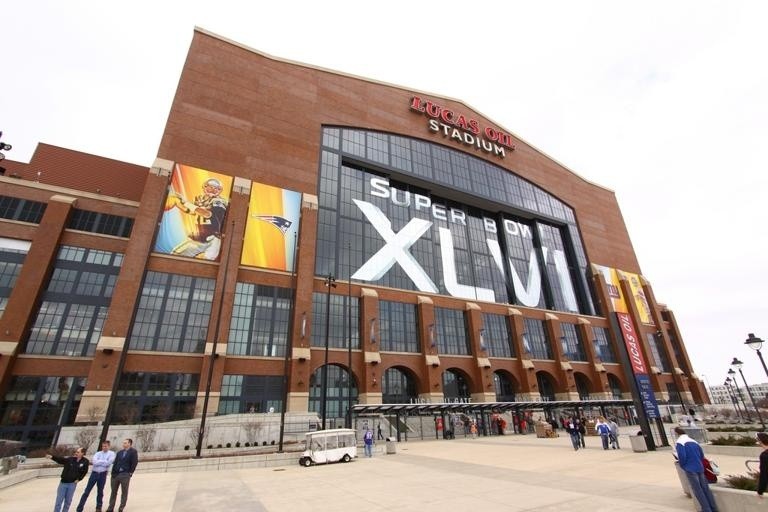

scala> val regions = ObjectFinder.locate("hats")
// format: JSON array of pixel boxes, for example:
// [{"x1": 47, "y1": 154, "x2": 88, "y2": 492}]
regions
[{"x1": 756, "y1": 431, "x2": 768, "y2": 446}]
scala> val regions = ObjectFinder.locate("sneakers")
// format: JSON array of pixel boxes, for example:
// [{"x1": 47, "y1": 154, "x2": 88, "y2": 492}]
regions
[
  {"x1": 105, "y1": 510, "x2": 114, "y2": 512},
  {"x1": 575, "y1": 445, "x2": 585, "y2": 451},
  {"x1": 96, "y1": 507, "x2": 101, "y2": 512},
  {"x1": 603, "y1": 446, "x2": 621, "y2": 450}
]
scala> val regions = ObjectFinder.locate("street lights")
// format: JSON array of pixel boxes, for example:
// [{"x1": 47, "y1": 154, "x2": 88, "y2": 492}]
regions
[
  {"x1": 700, "y1": 374, "x2": 717, "y2": 403},
  {"x1": 723, "y1": 332, "x2": 768, "y2": 432},
  {"x1": 320, "y1": 271, "x2": 338, "y2": 432}
]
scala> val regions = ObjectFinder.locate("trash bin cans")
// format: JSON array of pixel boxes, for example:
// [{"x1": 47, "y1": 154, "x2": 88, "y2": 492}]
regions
[
  {"x1": 629, "y1": 435, "x2": 648, "y2": 453},
  {"x1": 386, "y1": 436, "x2": 396, "y2": 454},
  {"x1": 675, "y1": 461, "x2": 692, "y2": 498}
]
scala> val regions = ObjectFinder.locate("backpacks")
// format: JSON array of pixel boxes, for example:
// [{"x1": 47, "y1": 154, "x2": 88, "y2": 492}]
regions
[
  {"x1": 599, "y1": 424, "x2": 607, "y2": 433},
  {"x1": 703, "y1": 457, "x2": 721, "y2": 475},
  {"x1": 579, "y1": 426, "x2": 586, "y2": 433}
]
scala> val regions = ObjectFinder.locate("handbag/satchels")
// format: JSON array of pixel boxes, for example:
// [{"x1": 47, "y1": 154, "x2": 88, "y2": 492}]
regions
[{"x1": 609, "y1": 434, "x2": 616, "y2": 442}]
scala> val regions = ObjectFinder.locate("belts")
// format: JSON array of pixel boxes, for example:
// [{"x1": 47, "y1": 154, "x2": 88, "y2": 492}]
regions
[
  {"x1": 92, "y1": 470, "x2": 108, "y2": 474},
  {"x1": 118, "y1": 470, "x2": 127, "y2": 474}
]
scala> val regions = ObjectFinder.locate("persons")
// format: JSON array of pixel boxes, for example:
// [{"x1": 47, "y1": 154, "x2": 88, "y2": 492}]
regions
[
  {"x1": 363, "y1": 430, "x2": 373, "y2": 457},
  {"x1": 756, "y1": 433, "x2": 768, "y2": 495},
  {"x1": 498, "y1": 417, "x2": 507, "y2": 435},
  {"x1": 674, "y1": 427, "x2": 718, "y2": 512},
  {"x1": 559, "y1": 414, "x2": 621, "y2": 451},
  {"x1": 470, "y1": 423, "x2": 478, "y2": 439},
  {"x1": 105, "y1": 438, "x2": 138, "y2": 512},
  {"x1": 76, "y1": 440, "x2": 116, "y2": 512},
  {"x1": 44, "y1": 447, "x2": 89, "y2": 512},
  {"x1": 170, "y1": 178, "x2": 228, "y2": 262}
]
